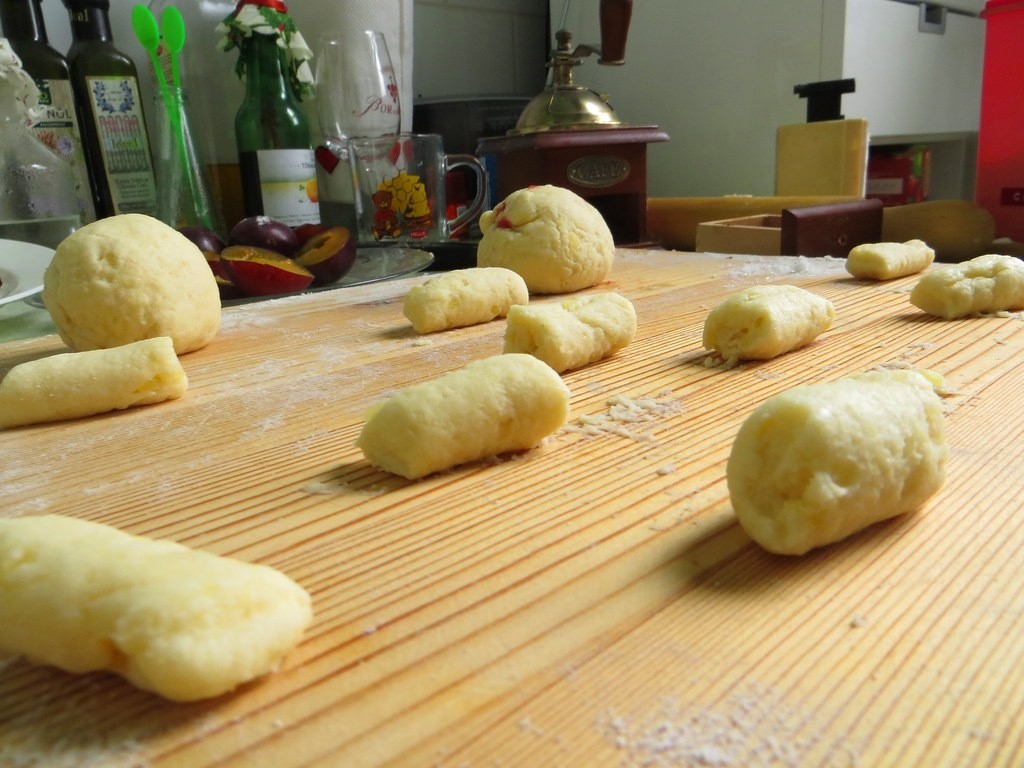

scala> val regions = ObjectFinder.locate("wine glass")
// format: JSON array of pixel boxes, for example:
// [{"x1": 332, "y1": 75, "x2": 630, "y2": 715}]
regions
[{"x1": 313, "y1": 30, "x2": 403, "y2": 247}]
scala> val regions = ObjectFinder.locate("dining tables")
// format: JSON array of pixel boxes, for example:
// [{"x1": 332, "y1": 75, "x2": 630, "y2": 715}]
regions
[{"x1": 0, "y1": 247, "x2": 1024, "y2": 768}]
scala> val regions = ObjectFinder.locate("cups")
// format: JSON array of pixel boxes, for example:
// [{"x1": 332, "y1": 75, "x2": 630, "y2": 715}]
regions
[{"x1": 351, "y1": 132, "x2": 485, "y2": 244}]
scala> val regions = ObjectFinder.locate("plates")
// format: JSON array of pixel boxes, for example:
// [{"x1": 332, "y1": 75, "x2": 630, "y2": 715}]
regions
[
  {"x1": 1, "y1": 237, "x2": 54, "y2": 310},
  {"x1": 222, "y1": 247, "x2": 435, "y2": 307}
]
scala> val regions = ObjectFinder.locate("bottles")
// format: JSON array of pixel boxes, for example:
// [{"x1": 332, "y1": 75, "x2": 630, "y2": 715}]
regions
[
  {"x1": 61, "y1": 1, "x2": 157, "y2": 220},
  {"x1": 1, "y1": 1, "x2": 94, "y2": 226},
  {"x1": 148, "y1": 80, "x2": 230, "y2": 256}
]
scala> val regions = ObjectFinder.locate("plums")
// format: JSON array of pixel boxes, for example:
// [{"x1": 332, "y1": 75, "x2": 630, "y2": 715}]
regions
[{"x1": 175, "y1": 215, "x2": 360, "y2": 299}]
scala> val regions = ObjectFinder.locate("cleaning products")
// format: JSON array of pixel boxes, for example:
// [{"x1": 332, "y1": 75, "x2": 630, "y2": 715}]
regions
[{"x1": 0, "y1": 31, "x2": 80, "y2": 314}]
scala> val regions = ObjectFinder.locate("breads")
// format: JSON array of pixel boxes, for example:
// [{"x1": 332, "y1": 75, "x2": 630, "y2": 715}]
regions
[
  {"x1": 1, "y1": 211, "x2": 224, "y2": 429},
  {"x1": 357, "y1": 185, "x2": 1024, "y2": 554},
  {"x1": 0, "y1": 515, "x2": 312, "y2": 701}
]
